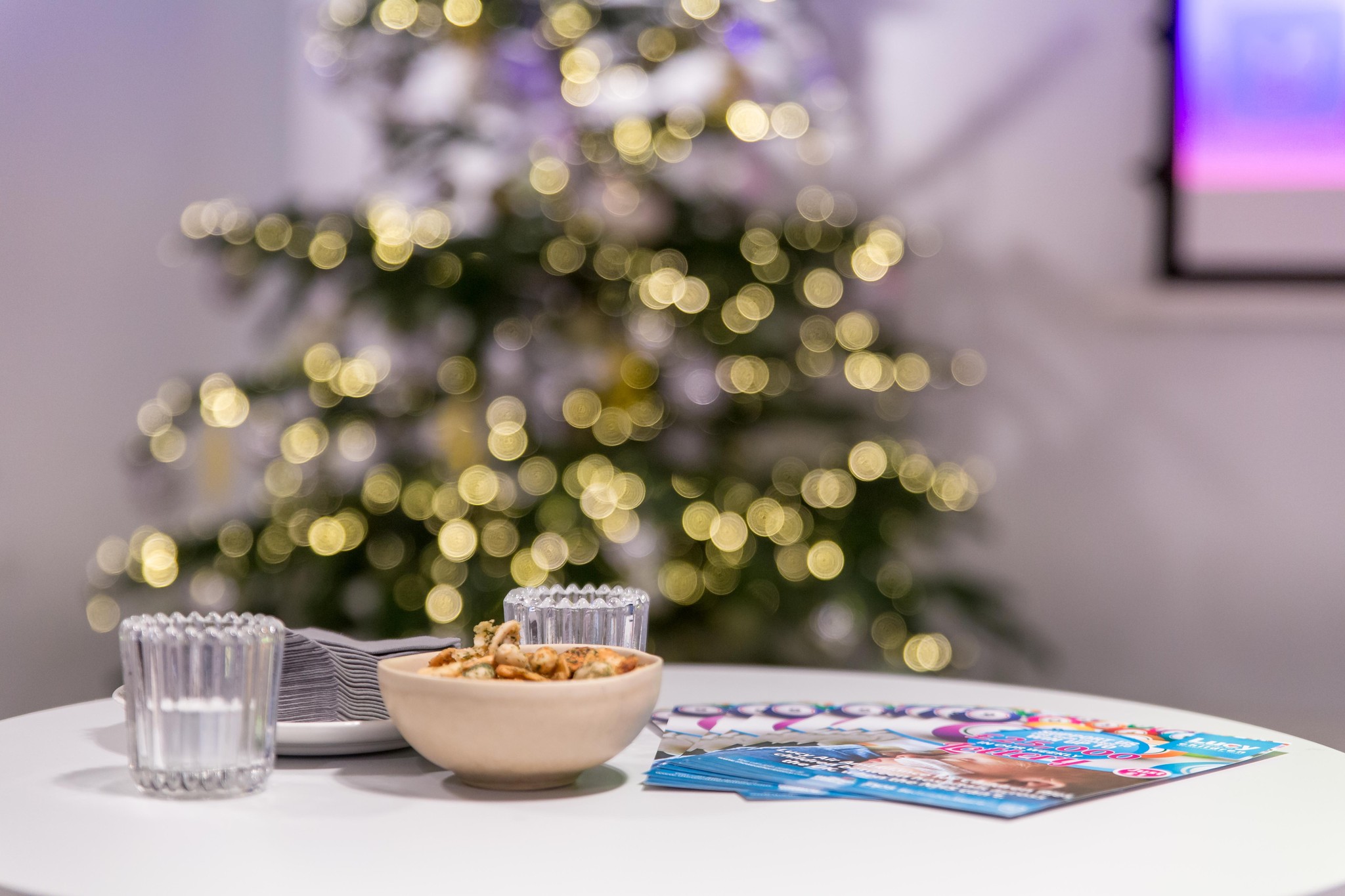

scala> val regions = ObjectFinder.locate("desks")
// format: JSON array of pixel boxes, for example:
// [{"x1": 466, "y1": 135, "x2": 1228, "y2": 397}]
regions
[{"x1": 0, "y1": 652, "x2": 1345, "y2": 896}]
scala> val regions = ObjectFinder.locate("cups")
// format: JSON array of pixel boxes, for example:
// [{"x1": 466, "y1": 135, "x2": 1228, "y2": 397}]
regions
[
  {"x1": 118, "y1": 612, "x2": 284, "y2": 795},
  {"x1": 503, "y1": 583, "x2": 650, "y2": 652}
]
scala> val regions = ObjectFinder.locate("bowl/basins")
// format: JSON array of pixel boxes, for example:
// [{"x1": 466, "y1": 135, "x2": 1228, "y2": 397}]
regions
[{"x1": 376, "y1": 643, "x2": 664, "y2": 791}]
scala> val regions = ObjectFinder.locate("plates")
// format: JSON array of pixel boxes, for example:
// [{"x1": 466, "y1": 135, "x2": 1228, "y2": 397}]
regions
[{"x1": 113, "y1": 675, "x2": 410, "y2": 755}]
